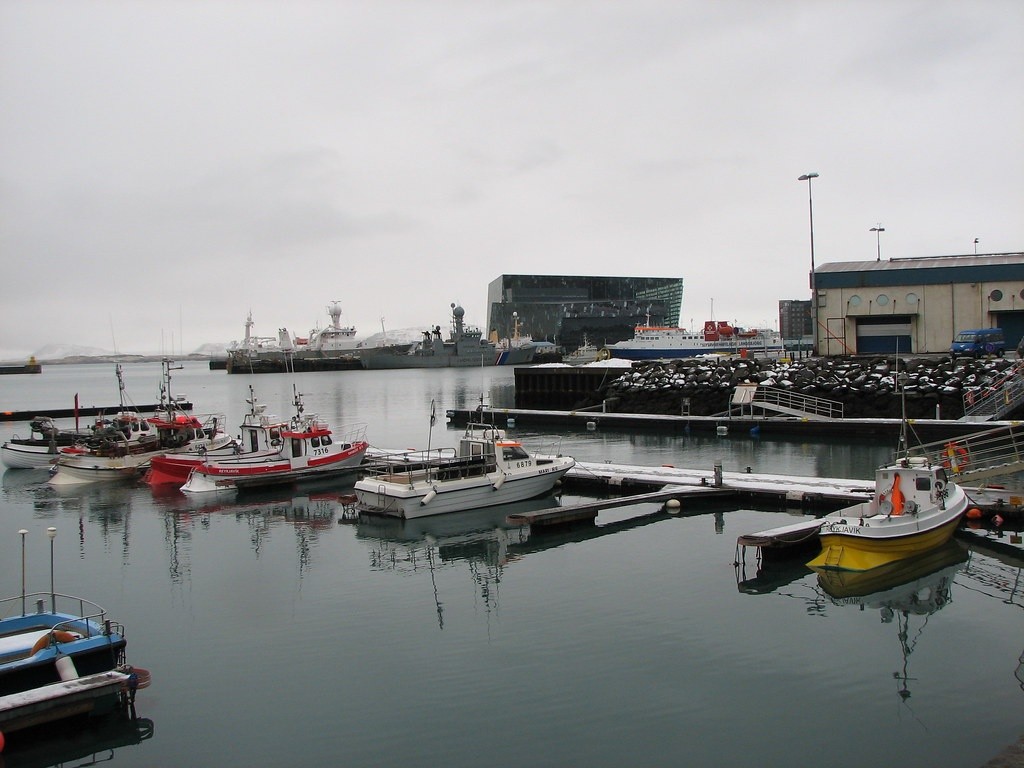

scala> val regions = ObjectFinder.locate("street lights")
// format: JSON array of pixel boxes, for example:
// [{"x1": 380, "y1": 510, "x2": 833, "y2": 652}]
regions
[
  {"x1": 869, "y1": 228, "x2": 886, "y2": 261},
  {"x1": 798, "y1": 173, "x2": 819, "y2": 356},
  {"x1": 974, "y1": 237, "x2": 979, "y2": 254}
]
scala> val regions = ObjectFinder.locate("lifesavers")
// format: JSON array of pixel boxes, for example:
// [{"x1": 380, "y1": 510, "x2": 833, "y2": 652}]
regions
[
  {"x1": 943, "y1": 447, "x2": 968, "y2": 470},
  {"x1": 30, "y1": 631, "x2": 76, "y2": 657},
  {"x1": 879, "y1": 488, "x2": 905, "y2": 515},
  {"x1": 599, "y1": 348, "x2": 611, "y2": 361}
]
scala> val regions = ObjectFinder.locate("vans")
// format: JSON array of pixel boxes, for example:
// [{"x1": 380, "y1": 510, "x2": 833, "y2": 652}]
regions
[
  {"x1": 1017, "y1": 336, "x2": 1024, "y2": 358},
  {"x1": 949, "y1": 328, "x2": 1005, "y2": 360}
]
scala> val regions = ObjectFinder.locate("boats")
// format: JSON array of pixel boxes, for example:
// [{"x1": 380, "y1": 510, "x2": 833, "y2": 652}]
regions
[
  {"x1": 354, "y1": 498, "x2": 565, "y2": 627},
  {"x1": 0, "y1": 527, "x2": 128, "y2": 697},
  {"x1": 144, "y1": 353, "x2": 291, "y2": 484},
  {"x1": 604, "y1": 298, "x2": 786, "y2": 364},
  {"x1": 562, "y1": 333, "x2": 606, "y2": 365},
  {"x1": 812, "y1": 542, "x2": 972, "y2": 702},
  {"x1": 180, "y1": 351, "x2": 369, "y2": 504},
  {"x1": 359, "y1": 302, "x2": 537, "y2": 370},
  {"x1": 339, "y1": 355, "x2": 576, "y2": 523},
  {"x1": 0, "y1": 312, "x2": 159, "y2": 470},
  {"x1": 49, "y1": 305, "x2": 243, "y2": 491},
  {"x1": 805, "y1": 386, "x2": 968, "y2": 583},
  {"x1": 227, "y1": 301, "x2": 413, "y2": 374}
]
